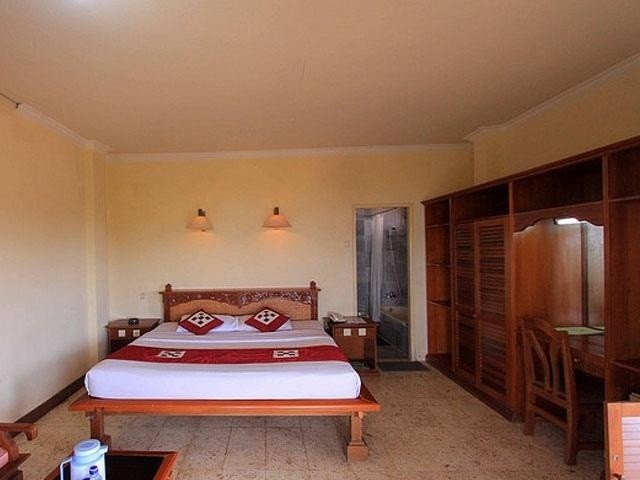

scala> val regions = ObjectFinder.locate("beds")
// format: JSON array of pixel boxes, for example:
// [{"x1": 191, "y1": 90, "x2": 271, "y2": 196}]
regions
[{"x1": 65, "y1": 280, "x2": 382, "y2": 463}]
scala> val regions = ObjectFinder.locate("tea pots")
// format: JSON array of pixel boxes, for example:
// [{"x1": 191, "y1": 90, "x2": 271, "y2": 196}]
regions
[{"x1": 59, "y1": 438, "x2": 108, "y2": 480}]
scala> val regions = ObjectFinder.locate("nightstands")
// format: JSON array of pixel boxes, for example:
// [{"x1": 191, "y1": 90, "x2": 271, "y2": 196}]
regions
[
  {"x1": 104, "y1": 317, "x2": 162, "y2": 355},
  {"x1": 322, "y1": 315, "x2": 382, "y2": 377}
]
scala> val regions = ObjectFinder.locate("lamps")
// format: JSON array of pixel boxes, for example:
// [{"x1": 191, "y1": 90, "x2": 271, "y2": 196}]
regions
[
  {"x1": 260, "y1": 205, "x2": 294, "y2": 230},
  {"x1": 185, "y1": 206, "x2": 215, "y2": 234}
]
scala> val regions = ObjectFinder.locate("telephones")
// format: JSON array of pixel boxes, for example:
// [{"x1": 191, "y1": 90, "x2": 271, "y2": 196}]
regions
[{"x1": 328, "y1": 311, "x2": 346, "y2": 323}]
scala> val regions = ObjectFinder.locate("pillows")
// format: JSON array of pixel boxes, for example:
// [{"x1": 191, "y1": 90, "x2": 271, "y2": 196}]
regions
[
  {"x1": 233, "y1": 315, "x2": 294, "y2": 332},
  {"x1": 243, "y1": 305, "x2": 292, "y2": 334},
  {"x1": 175, "y1": 315, "x2": 240, "y2": 335},
  {"x1": 178, "y1": 308, "x2": 226, "y2": 337}
]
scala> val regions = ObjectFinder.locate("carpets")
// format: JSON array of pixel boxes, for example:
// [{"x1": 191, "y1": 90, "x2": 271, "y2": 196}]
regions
[{"x1": 379, "y1": 359, "x2": 432, "y2": 373}]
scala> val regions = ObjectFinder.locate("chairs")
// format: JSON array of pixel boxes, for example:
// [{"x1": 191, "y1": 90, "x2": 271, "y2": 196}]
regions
[
  {"x1": 518, "y1": 315, "x2": 606, "y2": 467},
  {"x1": 0, "y1": 418, "x2": 40, "y2": 480}
]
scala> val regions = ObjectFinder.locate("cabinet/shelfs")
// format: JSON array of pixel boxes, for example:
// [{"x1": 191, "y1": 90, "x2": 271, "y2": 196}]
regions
[
  {"x1": 605, "y1": 137, "x2": 640, "y2": 479},
  {"x1": 419, "y1": 191, "x2": 453, "y2": 381},
  {"x1": 453, "y1": 148, "x2": 605, "y2": 425}
]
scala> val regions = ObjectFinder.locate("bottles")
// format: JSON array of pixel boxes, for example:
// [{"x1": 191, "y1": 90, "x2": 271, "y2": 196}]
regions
[{"x1": 88, "y1": 465, "x2": 104, "y2": 480}]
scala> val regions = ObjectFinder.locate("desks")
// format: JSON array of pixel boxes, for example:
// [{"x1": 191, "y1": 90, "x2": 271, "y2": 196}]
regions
[{"x1": 516, "y1": 325, "x2": 604, "y2": 384}]
